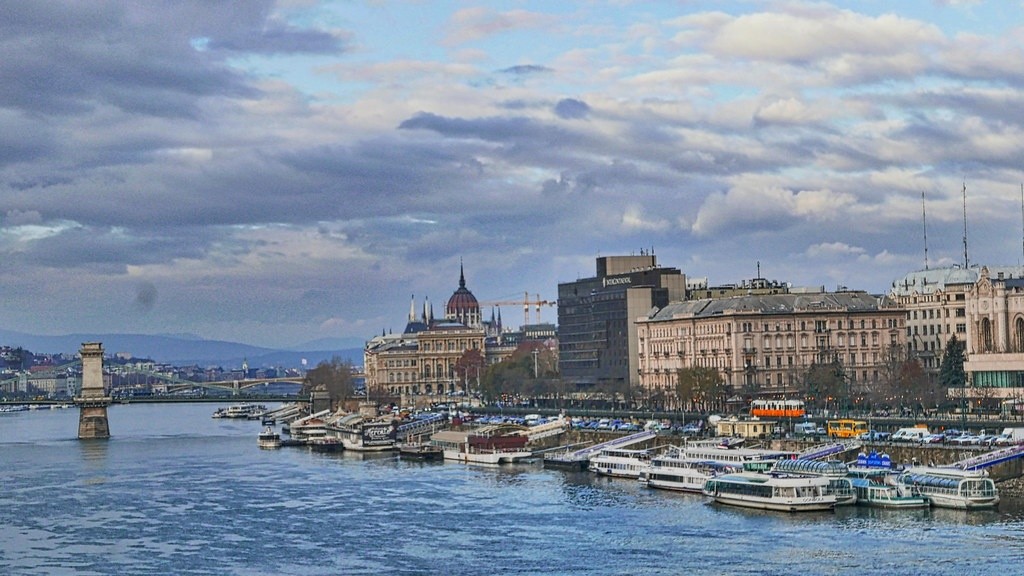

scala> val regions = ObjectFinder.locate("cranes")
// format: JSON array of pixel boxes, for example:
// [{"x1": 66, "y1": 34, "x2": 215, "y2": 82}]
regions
[{"x1": 444, "y1": 291, "x2": 557, "y2": 326}]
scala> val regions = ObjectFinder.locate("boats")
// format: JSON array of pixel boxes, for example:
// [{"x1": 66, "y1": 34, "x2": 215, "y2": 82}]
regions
[{"x1": 255, "y1": 426, "x2": 283, "y2": 450}]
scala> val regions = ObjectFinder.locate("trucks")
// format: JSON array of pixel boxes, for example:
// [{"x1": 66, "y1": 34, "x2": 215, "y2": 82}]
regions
[{"x1": 794, "y1": 424, "x2": 817, "y2": 437}]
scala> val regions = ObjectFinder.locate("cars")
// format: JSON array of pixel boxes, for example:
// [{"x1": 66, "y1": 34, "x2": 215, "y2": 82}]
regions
[
  {"x1": 210, "y1": 402, "x2": 1024, "y2": 515},
  {"x1": 428, "y1": 389, "x2": 487, "y2": 398}
]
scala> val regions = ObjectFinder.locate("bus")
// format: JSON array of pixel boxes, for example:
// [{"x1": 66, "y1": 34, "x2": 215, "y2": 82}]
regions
[
  {"x1": 827, "y1": 418, "x2": 869, "y2": 439},
  {"x1": 749, "y1": 399, "x2": 806, "y2": 417}
]
